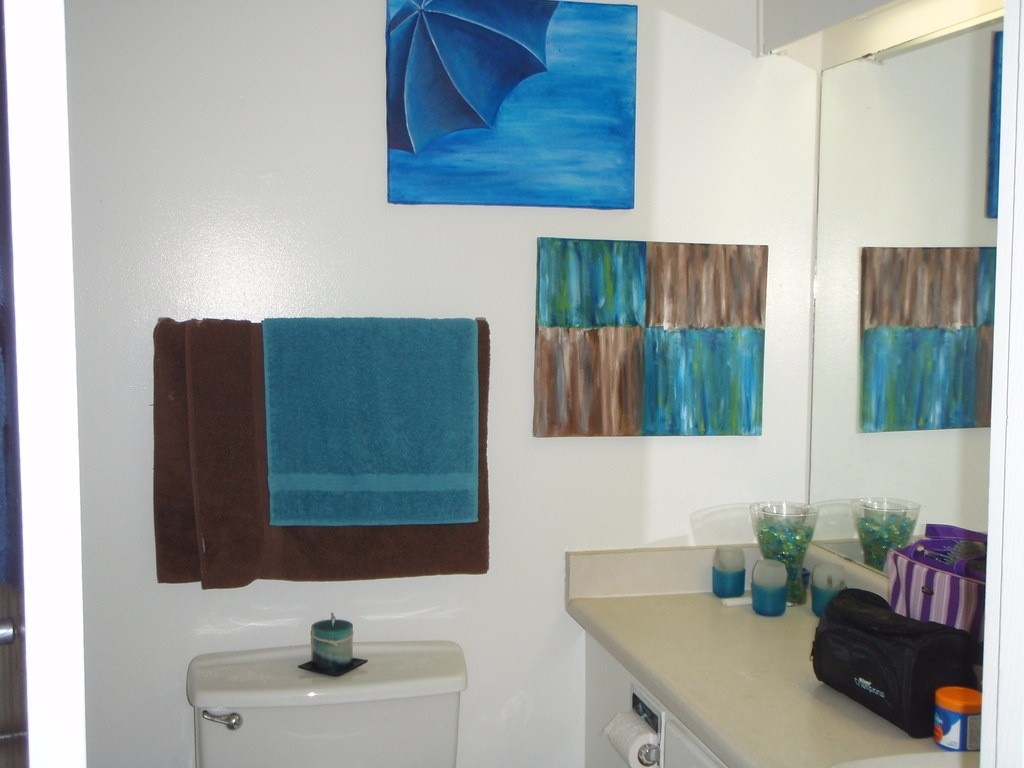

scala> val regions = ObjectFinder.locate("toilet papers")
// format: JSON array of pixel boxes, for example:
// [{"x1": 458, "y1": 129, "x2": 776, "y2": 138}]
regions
[{"x1": 601, "y1": 710, "x2": 659, "y2": 767}]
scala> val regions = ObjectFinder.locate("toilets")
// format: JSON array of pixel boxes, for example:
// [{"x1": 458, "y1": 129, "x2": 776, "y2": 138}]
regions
[{"x1": 189, "y1": 636, "x2": 474, "y2": 766}]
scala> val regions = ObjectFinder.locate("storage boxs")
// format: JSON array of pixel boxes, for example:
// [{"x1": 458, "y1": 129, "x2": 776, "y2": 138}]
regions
[
  {"x1": 881, "y1": 535, "x2": 989, "y2": 635},
  {"x1": 808, "y1": 587, "x2": 985, "y2": 745}
]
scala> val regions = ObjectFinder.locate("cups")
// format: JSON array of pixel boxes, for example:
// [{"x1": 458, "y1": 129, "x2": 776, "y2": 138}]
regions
[
  {"x1": 748, "y1": 501, "x2": 819, "y2": 607},
  {"x1": 810, "y1": 584, "x2": 848, "y2": 618},
  {"x1": 849, "y1": 497, "x2": 920, "y2": 571},
  {"x1": 751, "y1": 582, "x2": 788, "y2": 616},
  {"x1": 712, "y1": 566, "x2": 745, "y2": 598}
]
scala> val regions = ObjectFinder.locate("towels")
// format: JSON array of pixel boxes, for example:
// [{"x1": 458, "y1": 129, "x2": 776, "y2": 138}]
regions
[
  {"x1": 147, "y1": 316, "x2": 492, "y2": 591},
  {"x1": 258, "y1": 317, "x2": 481, "y2": 528}
]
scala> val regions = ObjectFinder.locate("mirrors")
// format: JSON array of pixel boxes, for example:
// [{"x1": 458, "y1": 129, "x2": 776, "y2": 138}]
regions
[{"x1": 799, "y1": 14, "x2": 1011, "y2": 586}]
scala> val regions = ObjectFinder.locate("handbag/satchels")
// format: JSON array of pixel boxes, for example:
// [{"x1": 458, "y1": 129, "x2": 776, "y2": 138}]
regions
[
  {"x1": 809, "y1": 587, "x2": 984, "y2": 739},
  {"x1": 887, "y1": 539, "x2": 987, "y2": 651},
  {"x1": 925, "y1": 524, "x2": 988, "y2": 538}
]
